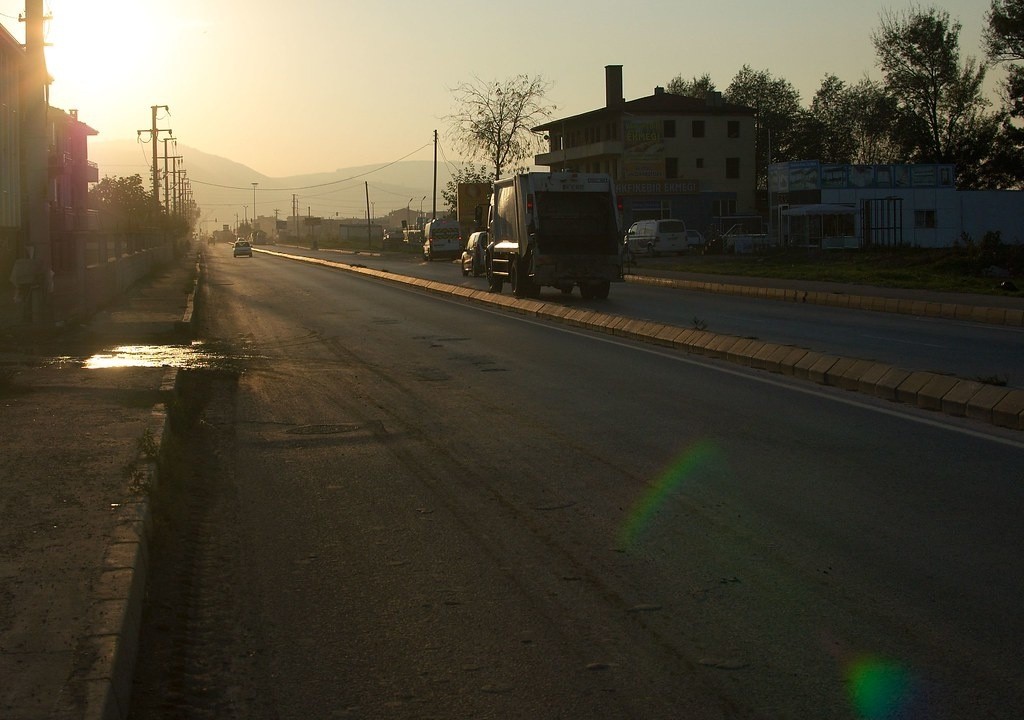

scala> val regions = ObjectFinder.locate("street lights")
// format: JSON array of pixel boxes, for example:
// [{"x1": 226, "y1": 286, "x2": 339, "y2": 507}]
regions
[
  {"x1": 252, "y1": 182, "x2": 259, "y2": 223},
  {"x1": 371, "y1": 202, "x2": 377, "y2": 221},
  {"x1": 420, "y1": 195, "x2": 427, "y2": 218},
  {"x1": 407, "y1": 198, "x2": 412, "y2": 227}
]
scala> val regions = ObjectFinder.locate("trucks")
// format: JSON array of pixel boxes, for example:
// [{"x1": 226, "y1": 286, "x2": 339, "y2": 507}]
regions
[{"x1": 474, "y1": 167, "x2": 625, "y2": 304}]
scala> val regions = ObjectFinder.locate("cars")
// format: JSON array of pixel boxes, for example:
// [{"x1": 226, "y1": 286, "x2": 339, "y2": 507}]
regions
[
  {"x1": 685, "y1": 229, "x2": 707, "y2": 256},
  {"x1": 460, "y1": 230, "x2": 489, "y2": 279},
  {"x1": 232, "y1": 240, "x2": 253, "y2": 258}
]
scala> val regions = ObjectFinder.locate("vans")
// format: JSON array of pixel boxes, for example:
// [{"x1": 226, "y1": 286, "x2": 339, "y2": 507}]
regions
[
  {"x1": 628, "y1": 217, "x2": 689, "y2": 258},
  {"x1": 423, "y1": 216, "x2": 464, "y2": 262}
]
